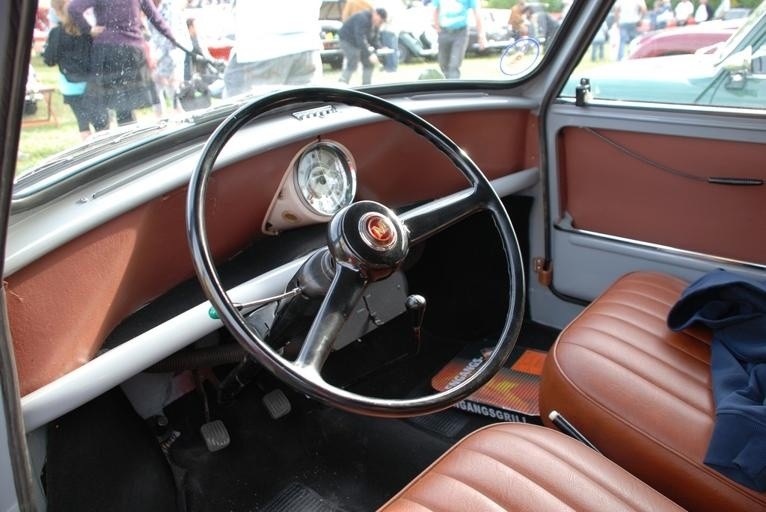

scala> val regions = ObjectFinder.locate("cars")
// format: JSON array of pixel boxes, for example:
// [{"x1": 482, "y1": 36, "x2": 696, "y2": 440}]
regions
[{"x1": 553, "y1": 0, "x2": 766, "y2": 116}]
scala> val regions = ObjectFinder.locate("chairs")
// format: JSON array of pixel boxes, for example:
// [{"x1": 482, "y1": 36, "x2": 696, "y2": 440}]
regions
[
  {"x1": 539, "y1": 271, "x2": 765, "y2": 511},
  {"x1": 378, "y1": 421, "x2": 686, "y2": 511}
]
